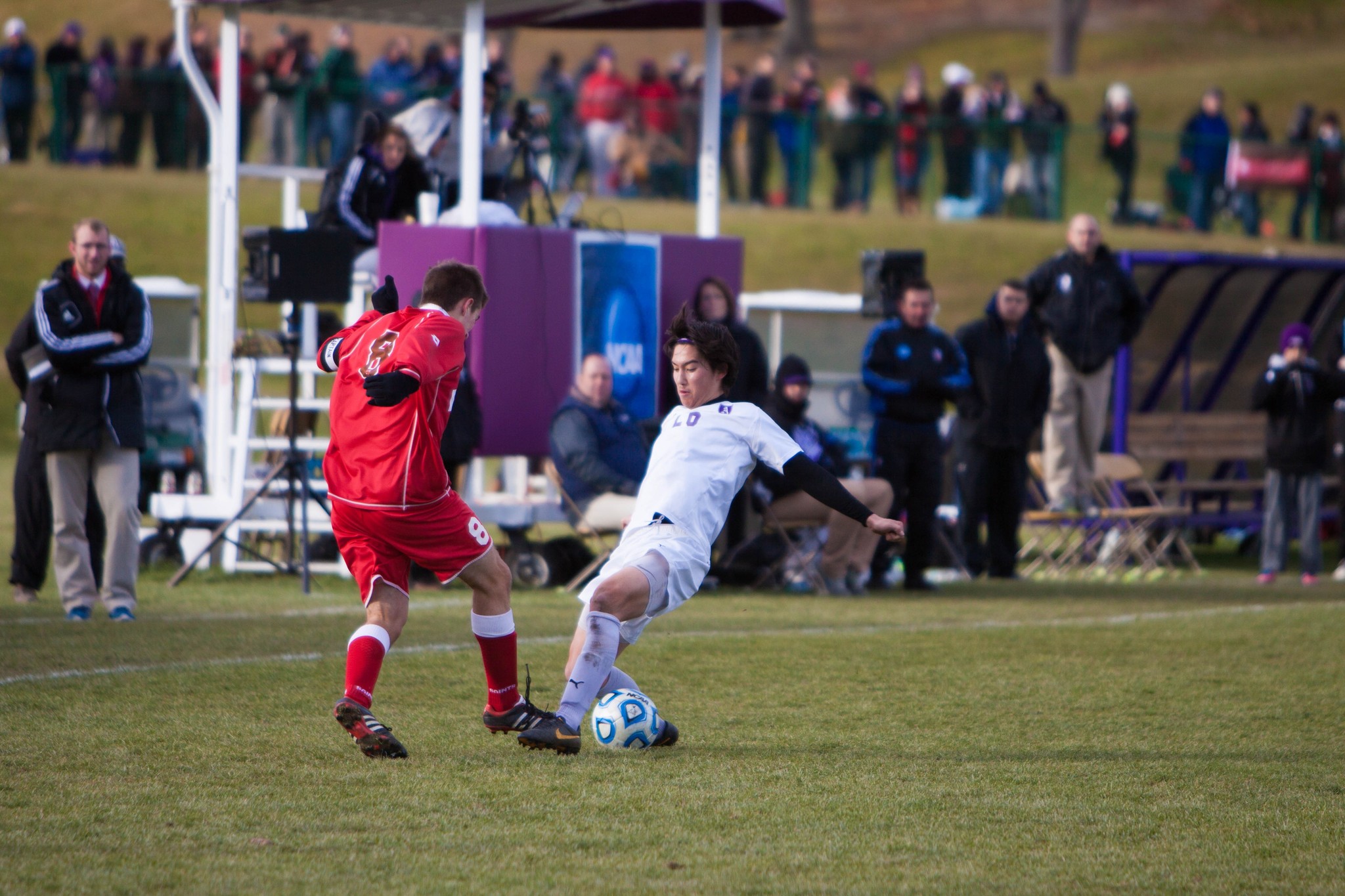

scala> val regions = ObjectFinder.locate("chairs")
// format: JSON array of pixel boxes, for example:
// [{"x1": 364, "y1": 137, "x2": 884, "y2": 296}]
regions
[{"x1": 538, "y1": 451, "x2": 1206, "y2": 596}]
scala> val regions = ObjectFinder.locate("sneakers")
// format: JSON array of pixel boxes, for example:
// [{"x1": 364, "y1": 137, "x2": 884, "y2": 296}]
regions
[
  {"x1": 517, "y1": 709, "x2": 581, "y2": 754},
  {"x1": 482, "y1": 662, "x2": 558, "y2": 734},
  {"x1": 653, "y1": 719, "x2": 678, "y2": 746},
  {"x1": 333, "y1": 698, "x2": 407, "y2": 759}
]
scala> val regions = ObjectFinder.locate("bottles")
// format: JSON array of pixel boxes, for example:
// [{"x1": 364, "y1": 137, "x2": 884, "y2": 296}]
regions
[
  {"x1": 186, "y1": 466, "x2": 202, "y2": 495},
  {"x1": 161, "y1": 466, "x2": 176, "y2": 494}
]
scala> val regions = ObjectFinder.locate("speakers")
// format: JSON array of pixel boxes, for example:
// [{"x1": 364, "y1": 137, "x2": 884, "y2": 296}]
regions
[
  {"x1": 240, "y1": 225, "x2": 354, "y2": 303},
  {"x1": 861, "y1": 250, "x2": 925, "y2": 316}
]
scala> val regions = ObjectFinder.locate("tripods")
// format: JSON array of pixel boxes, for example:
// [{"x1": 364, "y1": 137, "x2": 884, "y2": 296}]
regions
[
  {"x1": 490, "y1": 139, "x2": 561, "y2": 226},
  {"x1": 168, "y1": 305, "x2": 336, "y2": 594}
]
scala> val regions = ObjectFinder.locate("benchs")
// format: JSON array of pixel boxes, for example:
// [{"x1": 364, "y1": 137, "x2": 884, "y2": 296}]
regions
[{"x1": 1124, "y1": 411, "x2": 1345, "y2": 554}]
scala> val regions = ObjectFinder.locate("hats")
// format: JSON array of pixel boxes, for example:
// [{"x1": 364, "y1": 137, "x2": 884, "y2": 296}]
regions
[
  {"x1": 1280, "y1": 326, "x2": 1314, "y2": 353},
  {"x1": 108, "y1": 233, "x2": 125, "y2": 258}
]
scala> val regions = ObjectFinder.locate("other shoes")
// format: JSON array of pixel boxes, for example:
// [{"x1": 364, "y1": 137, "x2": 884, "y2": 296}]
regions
[
  {"x1": 1302, "y1": 571, "x2": 1314, "y2": 584},
  {"x1": 68, "y1": 606, "x2": 90, "y2": 622},
  {"x1": 848, "y1": 573, "x2": 868, "y2": 594},
  {"x1": 826, "y1": 575, "x2": 848, "y2": 597},
  {"x1": 13, "y1": 582, "x2": 39, "y2": 605},
  {"x1": 1257, "y1": 572, "x2": 1274, "y2": 584},
  {"x1": 110, "y1": 606, "x2": 134, "y2": 622}
]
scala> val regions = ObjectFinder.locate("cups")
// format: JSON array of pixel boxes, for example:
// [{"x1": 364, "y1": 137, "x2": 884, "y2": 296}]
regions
[{"x1": 417, "y1": 191, "x2": 439, "y2": 226}]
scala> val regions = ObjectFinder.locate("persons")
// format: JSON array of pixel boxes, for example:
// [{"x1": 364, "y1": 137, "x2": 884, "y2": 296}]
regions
[
  {"x1": 551, "y1": 349, "x2": 654, "y2": 536},
  {"x1": 0, "y1": 0, "x2": 1345, "y2": 604},
  {"x1": 861, "y1": 271, "x2": 970, "y2": 592},
  {"x1": 1029, "y1": 209, "x2": 1143, "y2": 519},
  {"x1": 510, "y1": 298, "x2": 907, "y2": 763},
  {"x1": 951, "y1": 275, "x2": 1054, "y2": 582},
  {"x1": 1253, "y1": 321, "x2": 1334, "y2": 587},
  {"x1": 1176, "y1": 84, "x2": 1232, "y2": 234},
  {"x1": 1099, "y1": 78, "x2": 1140, "y2": 224},
  {"x1": 316, "y1": 258, "x2": 566, "y2": 760},
  {"x1": 37, "y1": 214, "x2": 152, "y2": 623}
]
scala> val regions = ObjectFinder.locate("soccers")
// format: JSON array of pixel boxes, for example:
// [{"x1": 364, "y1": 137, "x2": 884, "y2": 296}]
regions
[{"x1": 590, "y1": 688, "x2": 661, "y2": 750}]
